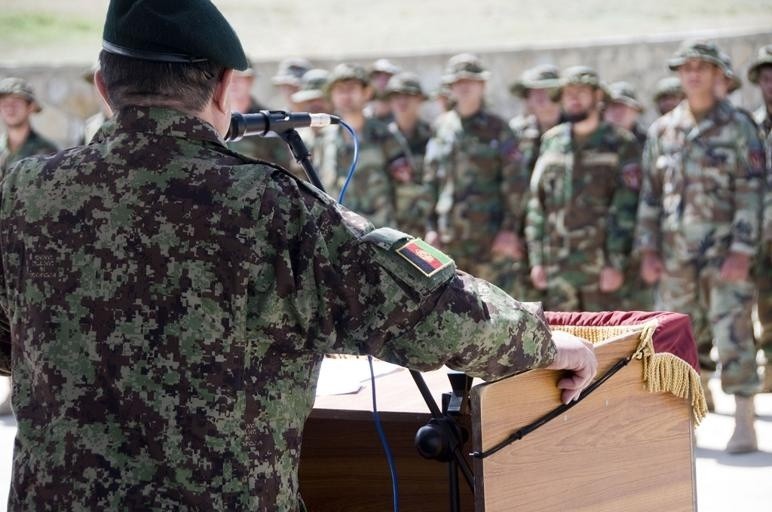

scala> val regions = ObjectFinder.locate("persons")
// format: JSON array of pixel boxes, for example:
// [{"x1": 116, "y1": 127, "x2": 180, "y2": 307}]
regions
[
  {"x1": 1, "y1": 59, "x2": 112, "y2": 416},
  {"x1": 0, "y1": 0, "x2": 598, "y2": 512},
  {"x1": 224, "y1": 40, "x2": 772, "y2": 453}
]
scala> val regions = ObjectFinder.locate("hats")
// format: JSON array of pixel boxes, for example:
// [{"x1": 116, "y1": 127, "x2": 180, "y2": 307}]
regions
[
  {"x1": 668, "y1": 41, "x2": 734, "y2": 80},
  {"x1": 440, "y1": 55, "x2": 486, "y2": 83},
  {"x1": 101, "y1": 0, "x2": 246, "y2": 72},
  {"x1": 274, "y1": 56, "x2": 426, "y2": 103},
  {"x1": 1, "y1": 78, "x2": 43, "y2": 114},
  {"x1": 607, "y1": 82, "x2": 643, "y2": 112},
  {"x1": 550, "y1": 68, "x2": 610, "y2": 102},
  {"x1": 720, "y1": 53, "x2": 741, "y2": 92},
  {"x1": 653, "y1": 81, "x2": 683, "y2": 101},
  {"x1": 510, "y1": 67, "x2": 562, "y2": 97},
  {"x1": 746, "y1": 45, "x2": 772, "y2": 84}
]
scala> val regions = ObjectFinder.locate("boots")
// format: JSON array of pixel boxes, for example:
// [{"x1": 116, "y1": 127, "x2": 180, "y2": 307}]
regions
[{"x1": 727, "y1": 394, "x2": 757, "y2": 453}]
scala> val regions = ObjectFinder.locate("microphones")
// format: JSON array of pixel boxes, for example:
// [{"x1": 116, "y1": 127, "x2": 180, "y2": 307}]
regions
[{"x1": 223, "y1": 109, "x2": 340, "y2": 143}]
